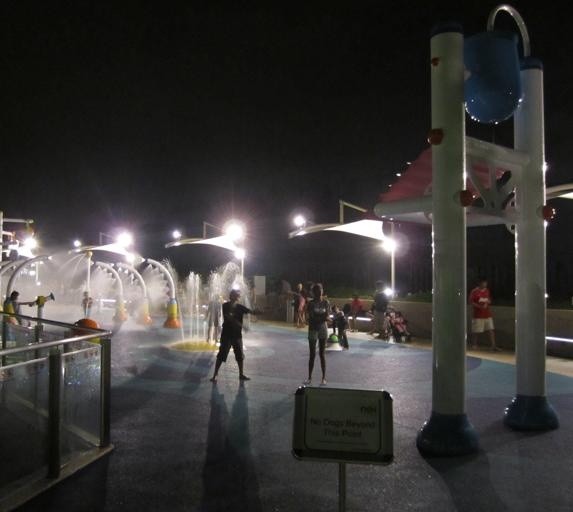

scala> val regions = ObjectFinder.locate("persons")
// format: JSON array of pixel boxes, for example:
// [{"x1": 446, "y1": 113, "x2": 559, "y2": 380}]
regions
[
  {"x1": 296, "y1": 281, "x2": 412, "y2": 349},
  {"x1": 469, "y1": 279, "x2": 502, "y2": 351},
  {"x1": 209, "y1": 290, "x2": 262, "y2": 382},
  {"x1": 202, "y1": 295, "x2": 221, "y2": 343},
  {"x1": 6, "y1": 291, "x2": 23, "y2": 326},
  {"x1": 82, "y1": 291, "x2": 92, "y2": 318},
  {"x1": 305, "y1": 284, "x2": 332, "y2": 386}
]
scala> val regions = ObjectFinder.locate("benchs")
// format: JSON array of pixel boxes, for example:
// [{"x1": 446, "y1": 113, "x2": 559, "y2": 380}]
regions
[
  {"x1": 327, "y1": 315, "x2": 372, "y2": 332},
  {"x1": 546, "y1": 336, "x2": 573, "y2": 358}
]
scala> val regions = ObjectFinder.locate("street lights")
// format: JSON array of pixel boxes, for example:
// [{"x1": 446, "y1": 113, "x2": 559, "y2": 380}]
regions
[
  {"x1": 164, "y1": 221, "x2": 244, "y2": 289},
  {"x1": 62, "y1": 230, "x2": 134, "y2": 283},
  {"x1": 287, "y1": 200, "x2": 397, "y2": 301},
  {"x1": 0, "y1": 210, "x2": 38, "y2": 271}
]
